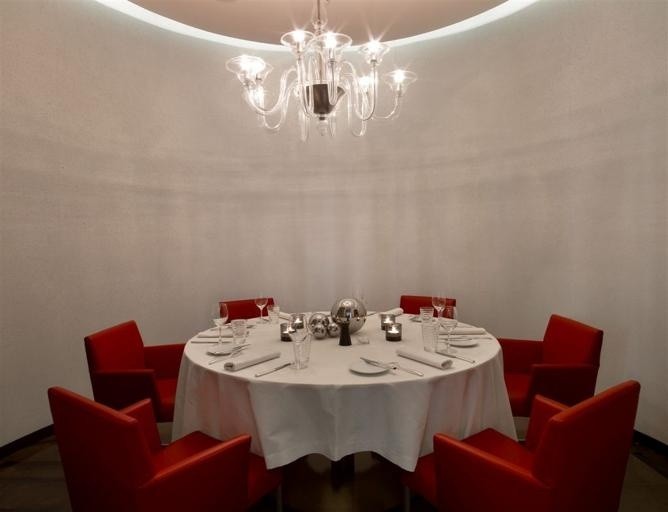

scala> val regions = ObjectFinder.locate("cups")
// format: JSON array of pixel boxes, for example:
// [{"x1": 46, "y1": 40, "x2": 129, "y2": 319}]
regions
[
  {"x1": 378, "y1": 313, "x2": 401, "y2": 341},
  {"x1": 420, "y1": 305, "x2": 436, "y2": 352}
]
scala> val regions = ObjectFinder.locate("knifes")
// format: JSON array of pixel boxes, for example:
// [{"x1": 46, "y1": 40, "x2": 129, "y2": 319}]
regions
[
  {"x1": 253, "y1": 362, "x2": 292, "y2": 378},
  {"x1": 436, "y1": 349, "x2": 474, "y2": 363}
]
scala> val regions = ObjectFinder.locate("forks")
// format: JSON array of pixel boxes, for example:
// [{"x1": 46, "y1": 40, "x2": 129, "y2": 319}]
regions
[{"x1": 390, "y1": 361, "x2": 424, "y2": 378}]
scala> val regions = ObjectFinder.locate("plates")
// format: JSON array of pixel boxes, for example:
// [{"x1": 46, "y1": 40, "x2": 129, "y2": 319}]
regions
[
  {"x1": 409, "y1": 316, "x2": 433, "y2": 323},
  {"x1": 447, "y1": 335, "x2": 477, "y2": 349},
  {"x1": 350, "y1": 358, "x2": 390, "y2": 374}
]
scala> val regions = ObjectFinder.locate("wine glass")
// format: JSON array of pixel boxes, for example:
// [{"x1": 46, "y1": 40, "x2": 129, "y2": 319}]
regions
[
  {"x1": 210, "y1": 292, "x2": 310, "y2": 370},
  {"x1": 431, "y1": 293, "x2": 458, "y2": 355}
]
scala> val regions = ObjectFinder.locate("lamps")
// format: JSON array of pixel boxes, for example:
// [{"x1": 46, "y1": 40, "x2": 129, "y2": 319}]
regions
[{"x1": 226, "y1": 0, "x2": 420, "y2": 142}]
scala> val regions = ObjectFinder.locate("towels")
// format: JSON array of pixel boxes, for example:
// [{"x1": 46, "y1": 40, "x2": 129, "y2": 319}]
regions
[
  {"x1": 379, "y1": 308, "x2": 405, "y2": 320},
  {"x1": 396, "y1": 345, "x2": 454, "y2": 371},
  {"x1": 224, "y1": 348, "x2": 281, "y2": 371},
  {"x1": 278, "y1": 311, "x2": 293, "y2": 320},
  {"x1": 436, "y1": 324, "x2": 487, "y2": 335},
  {"x1": 197, "y1": 330, "x2": 250, "y2": 337}
]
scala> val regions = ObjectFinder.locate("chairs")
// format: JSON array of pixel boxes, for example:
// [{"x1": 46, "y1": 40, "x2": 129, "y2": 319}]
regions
[
  {"x1": 399, "y1": 294, "x2": 456, "y2": 320},
  {"x1": 82, "y1": 320, "x2": 188, "y2": 422},
  {"x1": 404, "y1": 378, "x2": 640, "y2": 511},
  {"x1": 497, "y1": 312, "x2": 605, "y2": 416},
  {"x1": 48, "y1": 386, "x2": 284, "y2": 511},
  {"x1": 217, "y1": 296, "x2": 275, "y2": 323}
]
